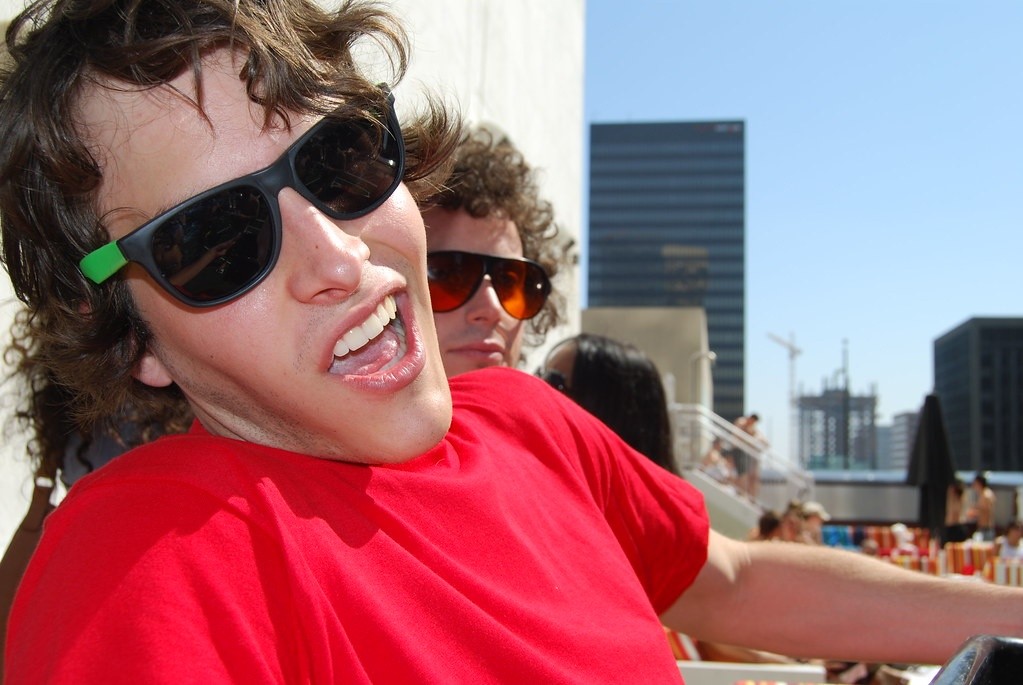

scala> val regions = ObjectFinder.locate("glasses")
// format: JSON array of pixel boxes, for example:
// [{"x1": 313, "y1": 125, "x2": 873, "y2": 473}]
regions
[
  {"x1": 80, "y1": 81, "x2": 409, "y2": 309},
  {"x1": 531, "y1": 367, "x2": 568, "y2": 397},
  {"x1": 424, "y1": 249, "x2": 553, "y2": 321}
]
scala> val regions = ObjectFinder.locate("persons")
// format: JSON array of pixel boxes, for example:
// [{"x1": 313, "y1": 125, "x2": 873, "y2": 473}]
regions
[
  {"x1": 993, "y1": 523, "x2": 1023, "y2": 558},
  {"x1": 846, "y1": 520, "x2": 917, "y2": 558},
  {"x1": 745, "y1": 500, "x2": 834, "y2": 546},
  {"x1": 0, "y1": 0, "x2": 1023, "y2": 685},
  {"x1": 942, "y1": 475, "x2": 997, "y2": 541},
  {"x1": 416, "y1": 124, "x2": 578, "y2": 378},
  {"x1": 704, "y1": 415, "x2": 770, "y2": 503},
  {"x1": 530, "y1": 333, "x2": 922, "y2": 685}
]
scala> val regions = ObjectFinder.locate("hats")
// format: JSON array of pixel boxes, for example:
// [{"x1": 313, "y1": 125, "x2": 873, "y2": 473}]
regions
[{"x1": 801, "y1": 501, "x2": 832, "y2": 522}]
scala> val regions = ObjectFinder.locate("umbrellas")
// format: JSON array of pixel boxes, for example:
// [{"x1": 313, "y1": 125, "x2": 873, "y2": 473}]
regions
[{"x1": 902, "y1": 395, "x2": 952, "y2": 537}]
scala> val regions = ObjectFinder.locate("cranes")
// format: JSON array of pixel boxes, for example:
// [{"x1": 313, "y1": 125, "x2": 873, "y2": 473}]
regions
[{"x1": 767, "y1": 329, "x2": 802, "y2": 467}]
